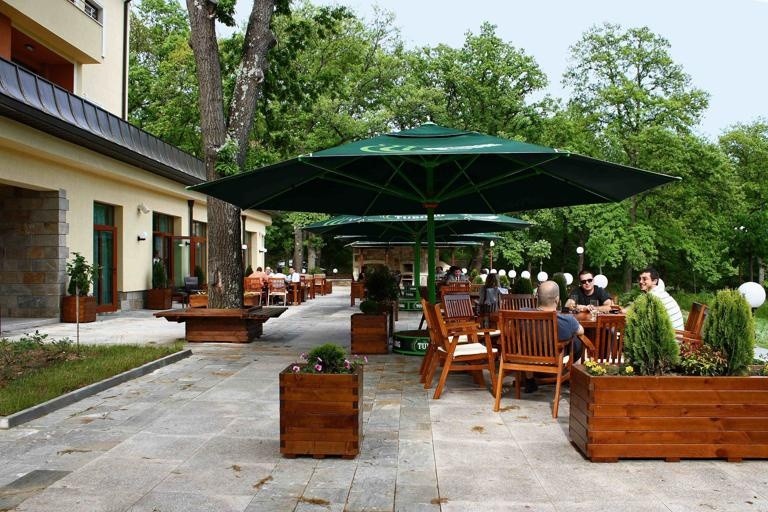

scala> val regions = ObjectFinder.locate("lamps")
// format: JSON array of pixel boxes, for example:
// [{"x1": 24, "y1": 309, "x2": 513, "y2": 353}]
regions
[
  {"x1": 137, "y1": 202, "x2": 149, "y2": 214},
  {"x1": 241, "y1": 243, "x2": 247, "y2": 251},
  {"x1": 137, "y1": 231, "x2": 147, "y2": 241}
]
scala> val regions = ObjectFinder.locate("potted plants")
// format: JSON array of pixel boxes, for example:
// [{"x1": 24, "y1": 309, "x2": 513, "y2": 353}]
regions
[
  {"x1": 350, "y1": 301, "x2": 388, "y2": 354},
  {"x1": 392, "y1": 273, "x2": 402, "y2": 321},
  {"x1": 362, "y1": 264, "x2": 395, "y2": 337},
  {"x1": 567, "y1": 288, "x2": 768, "y2": 462},
  {"x1": 144, "y1": 260, "x2": 172, "y2": 309},
  {"x1": 59, "y1": 252, "x2": 97, "y2": 324}
]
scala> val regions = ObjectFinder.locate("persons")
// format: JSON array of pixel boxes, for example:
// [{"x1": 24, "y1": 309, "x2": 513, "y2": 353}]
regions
[
  {"x1": 504, "y1": 281, "x2": 587, "y2": 394},
  {"x1": 472, "y1": 269, "x2": 488, "y2": 283},
  {"x1": 435, "y1": 266, "x2": 447, "y2": 280},
  {"x1": 248, "y1": 267, "x2": 268, "y2": 303},
  {"x1": 392, "y1": 270, "x2": 401, "y2": 286},
  {"x1": 447, "y1": 267, "x2": 468, "y2": 283},
  {"x1": 288, "y1": 264, "x2": 300, "y2": 283},
  {"x1": 271, "y1": 266, "x2": 291, "y2": 305},
  {"x1": 564, "y1": 269, "x2": 615, "y2": 312},
  {"x1": 357, "y1": 266, "x2": 369, "y2": 282},
  {"x1": 619, "y1": 269, "x2": 684, "y2": 345},
  {"x1": 478, "y1": 273, "x2": 503, "y2": 329},
  {"x1": 264, "y1": 267, "x2": 274, "y2": 276}
]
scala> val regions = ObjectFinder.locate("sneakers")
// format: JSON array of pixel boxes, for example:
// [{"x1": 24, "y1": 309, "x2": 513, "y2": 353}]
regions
[{"x1": 525, "y1": 379, "x2": 537, "y2": 393}]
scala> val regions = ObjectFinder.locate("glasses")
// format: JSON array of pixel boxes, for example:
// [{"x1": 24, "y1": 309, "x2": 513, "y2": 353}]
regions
[{"x1": 580, "y1": 279, "x2": 593, "y2": 283}]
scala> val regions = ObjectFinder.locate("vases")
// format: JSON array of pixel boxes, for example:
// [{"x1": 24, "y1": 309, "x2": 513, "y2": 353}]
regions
[{"x1": 279, "y1": 363, "x2": 364, "y2": 459}]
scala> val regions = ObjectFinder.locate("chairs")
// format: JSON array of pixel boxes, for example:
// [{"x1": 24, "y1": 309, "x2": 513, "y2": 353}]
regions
[
  {"x1": 419, "y1": 298, "x2": 481, "y2": 384},
  {"x1": 448, "y1": 281, "x2": 468, "y2": 287},
  {"x1": 468, "y1": 282, "x2": 484, "y2": 292},
  {"x1": 243, "y1": 276, "x2": 265, "y2": 306},
  {"x1": 473, "y1": 300, "x2": 498, "y2": 318},
  {"x1": 423, "y1": 302, "x2": 497, "y2": 399},
  {"x1": 576, "y1": 314, "x2": 626, "y2": 364},
  {"x1": 417, "y1": 286, "x2": 429, "y2": 330},
  {"x1": 493, "y1": 309, "x2": 573, "y2": 418},
  {"x1": 266, "y1": 278, "x2": 288, "y2": 306},
  {"x1": 441, "y1": 294, "x2": 474, "y2": 324},
  {"x1": 672, "y1": 302, "x2": 704, "y2": 335},
  {"x1": 674, "y1": 305, "x2": 710, "y2": 346},
  {"x1": 497, "y1": 292, "x2": 535, "y2": 310}
]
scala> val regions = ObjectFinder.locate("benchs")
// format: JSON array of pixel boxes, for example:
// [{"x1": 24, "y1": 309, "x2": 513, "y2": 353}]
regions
[{"x1": 153, "y1": 304, "x2": 288, "y2": 342}]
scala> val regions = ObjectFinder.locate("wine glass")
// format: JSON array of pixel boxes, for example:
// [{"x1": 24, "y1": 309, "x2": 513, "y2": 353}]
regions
[
  {"x1": 589, "y1": 299, "x2": 599, "y2": 321},
  {"x1": 570, "y1": 294, "x2": 579, "y2": 313}
]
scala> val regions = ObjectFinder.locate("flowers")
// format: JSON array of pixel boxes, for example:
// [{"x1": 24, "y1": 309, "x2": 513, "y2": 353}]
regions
[
  {"x1": 602, "y1": 361, "x2": 618, "y2": 375},
  {"x1": 619, "y1": 363, "x2": 635, "y2": 376},
  {"x1": 582, "y1": 360, "x2": 605, "y2": 377},
  {"x1": 291, "y1": 341, "x2": 367, "y2": 375}
]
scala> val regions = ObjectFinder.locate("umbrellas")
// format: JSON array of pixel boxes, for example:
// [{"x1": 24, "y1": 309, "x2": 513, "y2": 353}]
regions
[
  {"x1": 298, "y1": 211, "x2": 537, "y2": 301},
  {"x1": 184, "y1": 115, "x2": 683, "y2": 336},
  {"x1": 333, "y1": 231, "x2": 507, "y2": 300}
]
